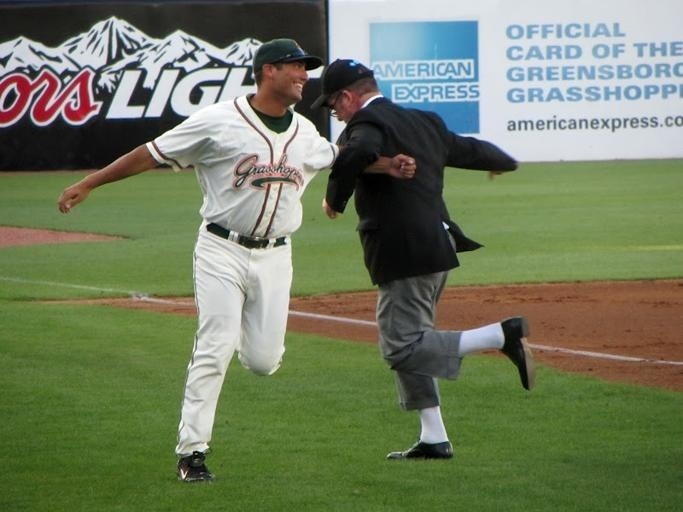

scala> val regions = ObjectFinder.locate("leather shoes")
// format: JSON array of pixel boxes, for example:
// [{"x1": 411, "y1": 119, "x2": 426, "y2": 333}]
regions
[
  {"x1": 501, "y1": 314, "x2": 536, "y2": 390},
  {"x1": 385, "y1": 438, "x2": 454, "y2": 461}
]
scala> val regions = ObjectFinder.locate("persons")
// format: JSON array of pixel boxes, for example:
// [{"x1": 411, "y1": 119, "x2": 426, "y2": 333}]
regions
[
  {"x1": 56, "y1": 36, "x2": 417, "y2": 484},
  {"x1": 310, "y1": 60, "x2": 538, "y2": 461}
]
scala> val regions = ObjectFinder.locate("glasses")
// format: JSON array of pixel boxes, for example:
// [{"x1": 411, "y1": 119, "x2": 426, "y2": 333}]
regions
[{"x1": 328, "y1": 90, "x2": 341, "y2": 117}]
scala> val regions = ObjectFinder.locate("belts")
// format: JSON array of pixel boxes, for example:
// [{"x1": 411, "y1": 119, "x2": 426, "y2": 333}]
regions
[{"x1": 206, "y1": 222, "x2": 286, "y2": 251}]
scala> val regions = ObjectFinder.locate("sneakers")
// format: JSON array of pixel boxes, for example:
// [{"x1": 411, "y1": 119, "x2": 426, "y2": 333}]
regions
[{"x1": 177, "y1": 450, "x2": 214, "y2": 483}]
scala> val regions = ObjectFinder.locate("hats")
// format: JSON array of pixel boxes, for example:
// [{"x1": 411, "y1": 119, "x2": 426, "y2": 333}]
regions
[
  {"x1": 310, "y1": 58, "x2": 375, "y2": 112},
  {"x1": 253, "y1": 38, "x2": 323, "y2": 71}
]
